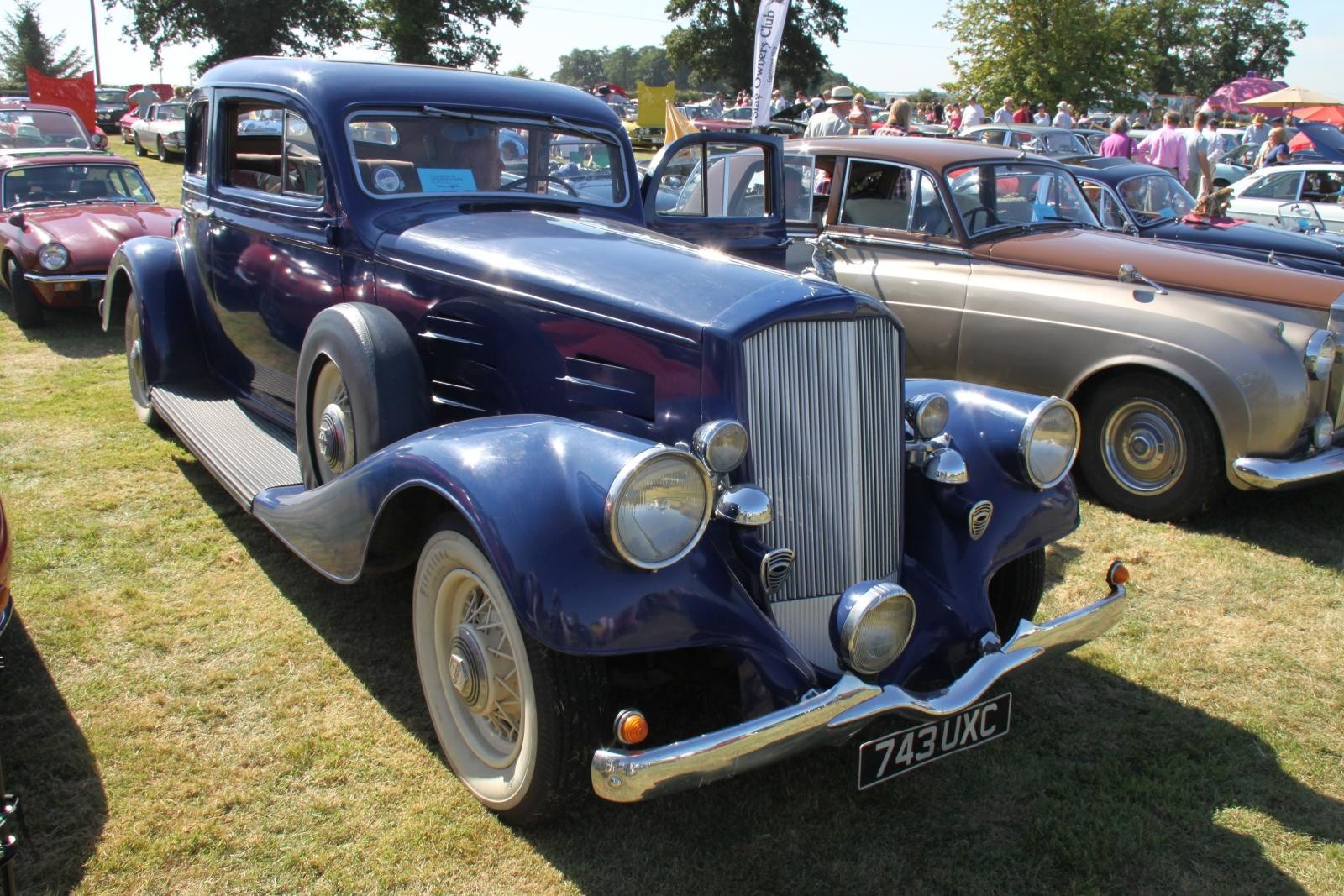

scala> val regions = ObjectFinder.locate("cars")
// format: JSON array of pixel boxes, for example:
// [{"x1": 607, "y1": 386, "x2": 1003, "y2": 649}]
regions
[
  {"x1": 0, "y1": 97, "x2": 108, "y2": 151},
  {"x1": 132, "y1": 102, "x2": 187, "y2": 162},
  {"x1": 673, "y1": 136, "x2": 1344, "y2": 523},
  {"x1": 119, "y1": 102, "x2": 155, "y2": 144},
  {"x1": 952, "y1": 153, "x2": 1344, "y2": 279},
  {"x1": 0, "y1": 153, "x2": 185, "y2": 328},
  {"x1": 97, "y1": 56, "x2": 1129, "y2": 825},
  {"x1": 1289, "y1": 121, "x2": 1344, "y2": 163},
  {"x1": 0, "y1": 500, "x2": 16, "y2": 638},
  {"x1": 1224, "y1": 163, "x2": 1344, "y2": 233},
  {"x1": 1212, "y1": 142, "x2": 1260, "y2": 192},
  {"x1": 95, "y1": 87, "x2": 129, "y2": 133},
  {"x1": 0, "y1": 103, "x2": 114, "y2": 156},
  {"x1": 238, "y1": 79, "x2": 1109, "y2": 210}
]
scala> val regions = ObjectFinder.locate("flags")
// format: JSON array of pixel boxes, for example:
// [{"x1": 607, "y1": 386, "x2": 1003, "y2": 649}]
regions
[{"x1": 751, "y1": 0, "x2": 789, "y2": 126}]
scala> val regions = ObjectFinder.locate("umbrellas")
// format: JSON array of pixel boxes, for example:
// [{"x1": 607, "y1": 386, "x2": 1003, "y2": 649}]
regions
[
  {"x1": 663, "y1": 100, "x2": 703, "y2": 163},
  {"x1": 1200, "y1": 76, "x2": 1344, "y2": 162}
]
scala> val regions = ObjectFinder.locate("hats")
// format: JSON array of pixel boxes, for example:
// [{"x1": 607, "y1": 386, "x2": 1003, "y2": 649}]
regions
[
  {"x1": 1206, "y1": 119, "x2": 1218, "y2": 124},
  {"x1": 966, "y1": 96, "x2": 975, "y2": 100},
  {"x1": 1037, "y1": 102, "x2": 1046, "y2": 108},
  {"x1": 773, "y1": 89, "x2": 780, "y2": 95},
  {"x1": 824, "y1": 85, "x2": 854, "y2": 104},
  {"x1": 1056, "y1": 100, "x2": 1067, "y2": 110}
]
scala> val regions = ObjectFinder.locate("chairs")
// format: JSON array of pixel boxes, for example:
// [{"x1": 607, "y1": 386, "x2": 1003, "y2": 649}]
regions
[
  {"x1": 844, "y1": 199, "x2": 910, "y2": 231},
  {"x1": 79, "y1": 179, "x2": 107, "y2": 200},
  {"x1": 932, "y1": 193, "x2": 987, "y2": 237}
]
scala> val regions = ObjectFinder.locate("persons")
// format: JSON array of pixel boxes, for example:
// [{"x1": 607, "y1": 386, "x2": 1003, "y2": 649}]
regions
[
  {"x1": 165, "y1": 86, "x2": 187, "y2": 119},
  {"x1": 129, "y1": 83, "x2": 162, "y2": 119},
  {"x1": 556, "y1": 134, "x2": 581, "y2": 159},
  {"x1": 734, "y1": 89, "x2": 786, "y2": 111},
  {"x1": 1013, "y1": 99, "x2": 1034, "y2": 143},
  {"x1": 712, "y1": 90, "x2": 723, "y2": 114},
  {"x1": 1186, "y1": 112, "x2": 1213, "y2": 198},
  {"x1": 1136, "y1": 110, "x2": 1188, "y2": 208},
  {"x1": 917, "y1": 96, "x2": 986, "y2": 137},
  {"x1": 885, "y1": 97, "x2": 895, "y2": 111},
  {"x1": 784, "y1": 167, "x2": 810, "y2": 220},
  {"x1": 607, "y1": 93, "x2": 627, "y2": 115},
  {"x1": 994, "y1": 96, "x2": 1014, "y2": 133},
  {"x1": 872, "y1": 98, "x2": 912, "y2": 200},
  {"x1": 1254, "y1": 126, "x2": 1289, "y2": 185},
  {"x1": 1099, "y1": 109, "x2": 1145, "y2": 199},
  {"x1": 844, "y1": 92, "x2": 870, "y2": 136},
  {"x1": 794, "y1": 89, "x2": 832, "y2": 122},
  {"x1": 803, "y1": 86, "x2": 856, "y2": 181},
  {"x1": 1197, "y1": 119, "x2": 1223, "y2": 201},
  {"x1": 1242, "y1": 112, "x2": 1273, "y2": 165},
  {"x1": 1033, "y1": 101, "x2": 1092, "y2": 130},
  {"x1": 451, "y1": 134, "x2": 507, "y2": 192}
]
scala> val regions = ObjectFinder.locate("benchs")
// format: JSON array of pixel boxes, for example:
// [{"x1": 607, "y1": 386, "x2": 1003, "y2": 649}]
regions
[
  {"x1": 234, "y1": 152, "x2": 416, "y2": 196},
  {"x1": 996, "y1": 197, "x2": 1031, "y2": 222}
]
